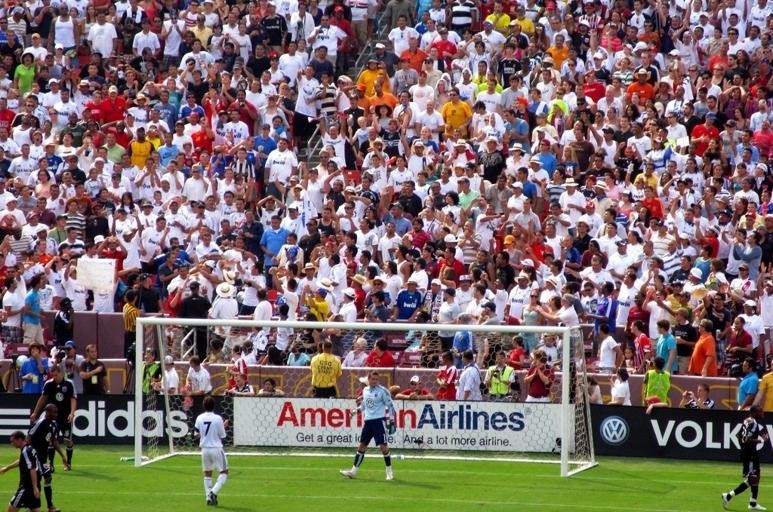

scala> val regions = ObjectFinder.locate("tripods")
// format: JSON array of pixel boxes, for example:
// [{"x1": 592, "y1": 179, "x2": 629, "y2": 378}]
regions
[{"x1": 6, "y1": 364, "x2": 20, "y2": 393}]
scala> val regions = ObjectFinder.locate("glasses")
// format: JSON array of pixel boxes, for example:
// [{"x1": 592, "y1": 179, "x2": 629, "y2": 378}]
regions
[
  {"x1": 198, "y1": 205, "x2": 204, "y2": 209},
  {"x1": 530, "y1": 294, "x2": 538, "y2": 298}
]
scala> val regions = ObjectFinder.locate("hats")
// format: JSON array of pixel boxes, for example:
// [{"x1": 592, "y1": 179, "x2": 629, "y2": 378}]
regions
[
  {"x1": 94, "y1": 234, "x2": 104, "y2": 244},
  {"x1": 481, "y1": 301, "x2": 496, "y2": 312},
  {"x1": 140, "y1": 201, "x2": 154, "y2": 208},
  {"x1": 414, "y1": 142, "x2": 425, "y2": 148},
  {"x1": 481, "y1": 20, "x2": 519, "y2": 50},
  {"x1": 743, "y1": 299, "x2": 756, "y2": 307},
  {"x1": 301, "y1": 261, "x2": 386, "y2": 299},
  {"x1": 59, "y1": 297, "x2": 73, "y2": 307},
  {"x1": 368, "y1": 137, "x2": 385, "y2": 149},
  {"x1": 374, "y1": 103, "x2": 401, "y2": 114},
  {"x1": 334, "y1": 6, "x2": 344, "y2": 12},
  {"x1": 656, "y1": 204, "x2": 732, "y2": 279},
  {"x1": 510, "y1": 154, "x2": 543, "y2": 189},
  {"x1": 594, "y1": 41, "x2": 680, "y2": 74},
  {"x1": 56, "y1": 214, "x2": 67, "y2": 221},
  {"x1": 451, "y1": 161, "x2": 476, "y2": 183},
  {"x1": 165, "y1": 355, "x2": 174, "y2": 365},
  {"x1": 454, "y1": 134, "x2": 528, "y2": 154},
  {"x1": 49, "y1": 78, "x2": 119, "y2": 95},
  {"x1": 20, "y1": 52, "x2": 34, "y2": 64},
  {"x1": 407, "y1": 273, "x2": 471, "y2": 296},
  {"x1": 706, "y1": 111, "x2": 716, "y2": 120},
  {"x1": 65, "y1": 341, "x2": 75, "y2": 350},
  {"x1": 737, "y1": 263, "x2": 749, "y2": 273},
  {"x1": 132, "y1": 94, "x2": 150, "y2": 105},
  {"x1": 189, "y1": 269, "x2": 236, "y2": 298},
  {"x1": 513, "y1": 258, "x2": 558, "y2": 289},
  {"x1": 354, "y1": 42, "x2": 386, "y2": 92},
  {"x1": 5, "y1": 195, "x2": 18, "y2": 205},
  {"x1": 573, "y1": 214, "x2": 594, "y2": 231},
  {"x1": 445, "y1": 233, "x2": 457, "y2": 244},
  {"x1": 409, "y1": 375, "x2": 419, "y2": 384},
  {"x1": 288, "y1": 174, "x2": 303, "y2": 211}
]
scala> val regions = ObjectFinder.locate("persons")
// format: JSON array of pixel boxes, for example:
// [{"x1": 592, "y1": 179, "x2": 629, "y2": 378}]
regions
[
  {"x1": 339, "y1": 370, "x2": 393, "y2": 480},
  {"x1": 26, "y1": 404, "x2": 72, "y2": 511},
  {"x1": 194, "y1": 396, "x2": 229, "y2": 507},
  {"x1": 2, "y1": 2, "x2": 773, "y2": 415},
  {"x1": 30, "y1": 365, "x2": 77, "y2": 474},
  {"x1": 2, "y1": 431, "x2": 43, "y2": 511},
  {"x1": 721, "y1": 404, "x2": 768, "y2": 511}
]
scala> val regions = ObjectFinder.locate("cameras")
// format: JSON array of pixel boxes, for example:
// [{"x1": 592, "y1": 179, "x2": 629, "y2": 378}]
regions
[
  {"x1": 493, "y1": 370, "x2": 500, "y2": 377},
  {"x1": 107, "y1": 237, "x2": 117, "y2": 243},
  {"x1": 725, "y1": 229, "x2": 731, "y2": 233},
  {"x1": 6, "y1": 352, "x2": 19, "y2": 359},
  {"x1": 687, "y1": 392, "x2": 692, "y2": 395},
  {"x1": 140, "y1": 273, "x2": 152, "y2": 280}
]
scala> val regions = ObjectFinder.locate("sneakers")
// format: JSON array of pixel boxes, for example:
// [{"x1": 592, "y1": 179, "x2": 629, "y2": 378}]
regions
[
  {"x1": 385, "y1": 463, "x2": 392, "y2": 481},
  {"x1": 339, "y1": 470, "x2": 356, "y2": 479},
  {"x1": 747, "y1": 502, "x2": 767, "y2": 510},
  {"x1": 48, "y1": 465, "x2": 55, "y2": 473},
  {"x1": 63, "y1": 463, "x2": 71, "y2": 471},
  {"x1": 206, "y1": 490, "x2": 218, "y2": 506},
  {"x1": 720, "y1": 492, "x2": 730, "y2": 510}
]
